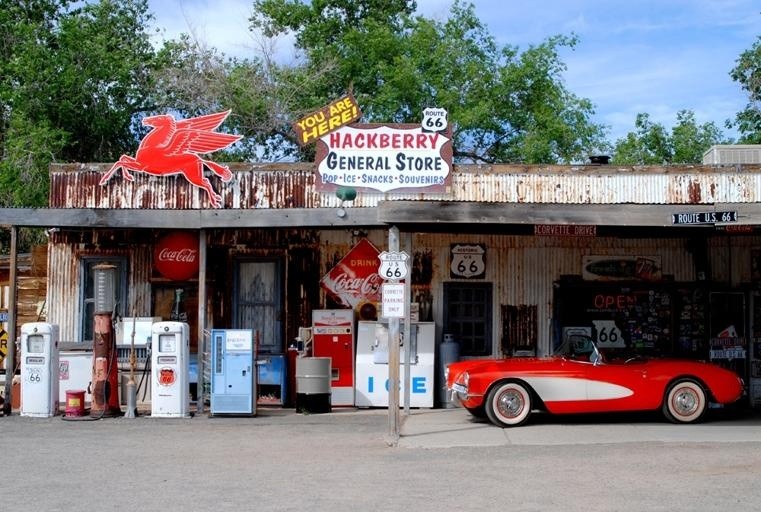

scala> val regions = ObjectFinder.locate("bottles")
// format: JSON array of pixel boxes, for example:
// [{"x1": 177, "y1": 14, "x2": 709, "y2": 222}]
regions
[{"x1": 170, "y1": 288, "x2": 190, "y2": 322}]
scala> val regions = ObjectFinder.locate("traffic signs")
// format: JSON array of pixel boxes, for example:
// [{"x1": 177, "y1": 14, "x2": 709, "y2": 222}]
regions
[
  {"x1": 378, "y1": 250, "x2": 409, "y2": 279},
  {"x1": 381, "y1": 282, "x2": 406, "y2": 318}
]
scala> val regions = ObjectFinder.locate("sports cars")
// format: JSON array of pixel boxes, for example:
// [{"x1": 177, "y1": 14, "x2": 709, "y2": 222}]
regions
[{"x1": 444, "y1": 334, "x2": 743, "y2": 427}]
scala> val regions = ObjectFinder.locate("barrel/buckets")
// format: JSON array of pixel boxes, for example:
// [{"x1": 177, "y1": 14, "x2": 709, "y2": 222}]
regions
[{"x1": 296, "y1": 357, "x2": 333, "y2": 413}]
